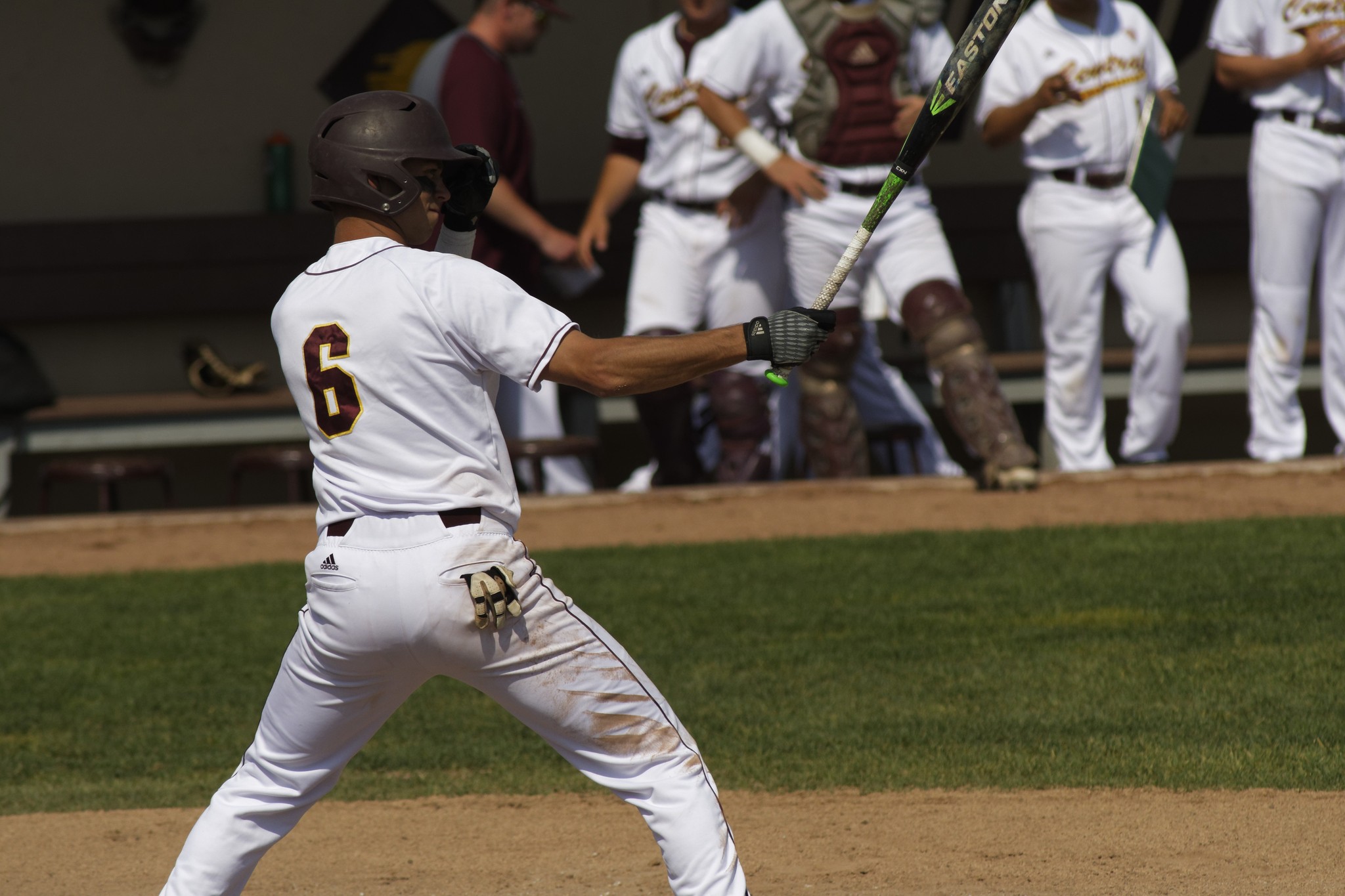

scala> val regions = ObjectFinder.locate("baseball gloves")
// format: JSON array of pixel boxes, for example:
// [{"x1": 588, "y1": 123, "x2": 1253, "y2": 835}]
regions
[
  {"x1": 434, "y1": 147, "x2": 496, "y2": 232},
  {"x1": 177, "y1": 337, "x2": 266, "y2": 395}
]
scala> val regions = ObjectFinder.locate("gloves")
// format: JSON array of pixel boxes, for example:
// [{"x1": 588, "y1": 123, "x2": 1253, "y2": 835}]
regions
[
  {"x1": 744, "y1": 306, "x2": 836, "y2": 366},
  {"x1": 461, "y1": 564, "x2": 522, "y2": 628}
]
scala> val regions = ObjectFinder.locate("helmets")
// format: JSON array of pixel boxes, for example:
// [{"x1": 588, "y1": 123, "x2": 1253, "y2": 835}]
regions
[{"x1": 305, "y1": 89, "x2": 482, "y2": 213}]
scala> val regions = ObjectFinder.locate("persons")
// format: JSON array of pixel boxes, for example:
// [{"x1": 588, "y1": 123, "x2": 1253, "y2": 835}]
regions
[
  {"x1": 407, "y1": 0, "x2": 1345, "y2": 496},
  {"x1": 157, "y1": 89, "x2": 830, "y2": 896},
  {"x1": 975, "y1": 0, "x2": 1192, "y2": 472},
  {"x1": 1208, "y1": 0, "x2": 1344, "y2": 462}
]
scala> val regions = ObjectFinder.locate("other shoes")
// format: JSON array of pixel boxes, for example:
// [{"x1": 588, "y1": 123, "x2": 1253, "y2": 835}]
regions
[{"x1": 997, "y1": 466, "x2": 1039, "y2": 489}]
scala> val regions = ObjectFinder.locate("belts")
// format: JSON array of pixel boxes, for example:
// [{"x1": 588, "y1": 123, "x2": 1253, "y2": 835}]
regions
[
  {"x1": 1053, "y1": 169, "x2": 1125, "y2": 188},
  {"x1": 1281, "y1": 109, "x2": 1345, "y2": 135},
  {"x1": 654, "y1": 192, "x2": 717, "y2": 211},
  {"x1": 841, "y1": 178, "x2": 915, "y2": 196},
  {"x1": 328, "y1": 506, "x2": 482, "y2": 536}
]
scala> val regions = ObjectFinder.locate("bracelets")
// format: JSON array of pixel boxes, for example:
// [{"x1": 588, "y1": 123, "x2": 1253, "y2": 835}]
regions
[{"x1": 736, "y1": 125, "x2": 781, "y2": 169}]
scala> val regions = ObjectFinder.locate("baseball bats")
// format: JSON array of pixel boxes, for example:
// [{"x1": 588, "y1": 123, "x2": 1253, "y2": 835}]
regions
[{"x1": 763, "y1": 1, "x2": 1034, "y2": 387}]
